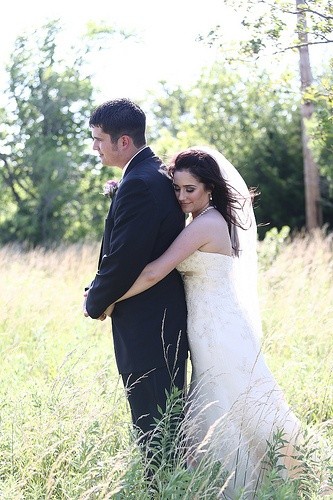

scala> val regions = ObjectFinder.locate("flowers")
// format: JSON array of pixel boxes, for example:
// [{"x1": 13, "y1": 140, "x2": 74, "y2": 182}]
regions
[{"x1": 99, "y1": 179, "x2": 119, "y2": 200}]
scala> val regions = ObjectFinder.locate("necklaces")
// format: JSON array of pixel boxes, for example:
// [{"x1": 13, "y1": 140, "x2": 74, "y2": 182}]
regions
[{"x1": 193, "y1": 206, "x2": 216, "y2": 221}]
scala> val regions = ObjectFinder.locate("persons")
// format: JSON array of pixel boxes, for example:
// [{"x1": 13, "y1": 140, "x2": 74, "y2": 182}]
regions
[
  {"x1": 83, "y1": 98, "x2": 189, "y2": 500},
  {"x1": 104, "y1": 145, "x2": 333, "y2": 500}
]
